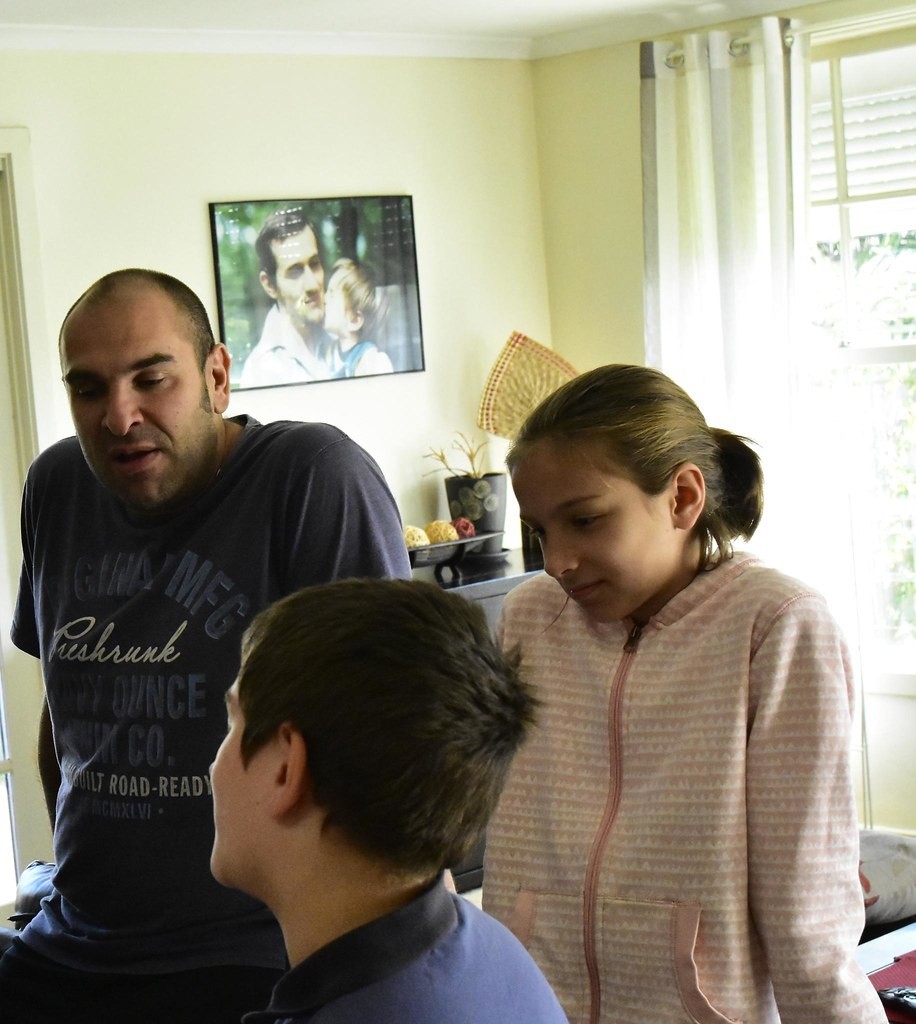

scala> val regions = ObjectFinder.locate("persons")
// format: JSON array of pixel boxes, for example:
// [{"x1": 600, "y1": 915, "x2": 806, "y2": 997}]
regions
[
  {"x1": 0, "y1": 271, "x2": 411, "y2": 1024},
  {"x1": 208, "y1": 581, "x2": 571, "y2": 1024},
  {"x1": 322, "y1": 257, "x2": 393, "y2": 379},
  {"x1": 482, "y1": 364, "x2": 890, "y2": 1024},
  {"x1": 240, "y1": 211, "x2": 339, "y2": 389}
]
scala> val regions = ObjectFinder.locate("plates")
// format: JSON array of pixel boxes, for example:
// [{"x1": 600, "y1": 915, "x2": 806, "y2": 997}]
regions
[{"x1": 407, "y1": 530, "x2": 505, "y2": 567}]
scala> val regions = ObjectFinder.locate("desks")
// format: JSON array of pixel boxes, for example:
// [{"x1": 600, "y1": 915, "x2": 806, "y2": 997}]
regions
[
  {"x1": 410, "y1": 547, "x2": 545, "y2": 643},
  {"x1": 858, "y1": 922, "x2": 916, "y2": 1024}
]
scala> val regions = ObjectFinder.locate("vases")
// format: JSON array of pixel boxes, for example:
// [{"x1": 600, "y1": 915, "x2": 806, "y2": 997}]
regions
[{"x1": 445, "y1": 473, "x2": 513, "y2": 564}]
